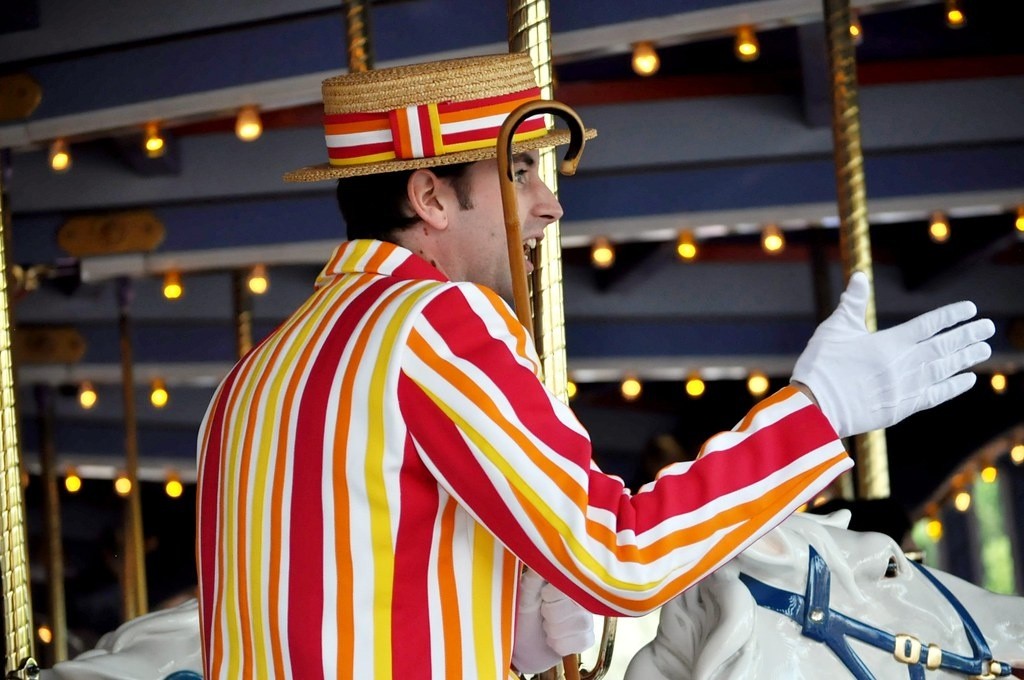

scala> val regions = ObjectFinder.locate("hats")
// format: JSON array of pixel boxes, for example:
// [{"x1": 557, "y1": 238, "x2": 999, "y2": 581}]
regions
[{"x1": 284, "y1": 52, "x2": 597, "y2": 182}]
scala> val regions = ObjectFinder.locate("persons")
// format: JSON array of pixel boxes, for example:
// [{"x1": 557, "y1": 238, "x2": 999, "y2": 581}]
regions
[{"x1": 197, "y1": 51, "x2": 998, "y2": 680}]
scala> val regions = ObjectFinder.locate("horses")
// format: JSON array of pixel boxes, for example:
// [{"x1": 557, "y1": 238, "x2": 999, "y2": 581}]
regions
[
  {"x1": 624, "y1": 509, "x2": 1024, "y2": 679},
  {"x1": 40, "y1": 595, "x2": 203, "y2": 679}
]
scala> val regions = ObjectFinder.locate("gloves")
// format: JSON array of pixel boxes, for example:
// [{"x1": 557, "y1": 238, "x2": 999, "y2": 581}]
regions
[
  {"x1": 512, "y1": 566, "x2": 595, "y2": 674},
  {"x1": 790, "y1": 272, "x2": 995, "y2": 439}
]
